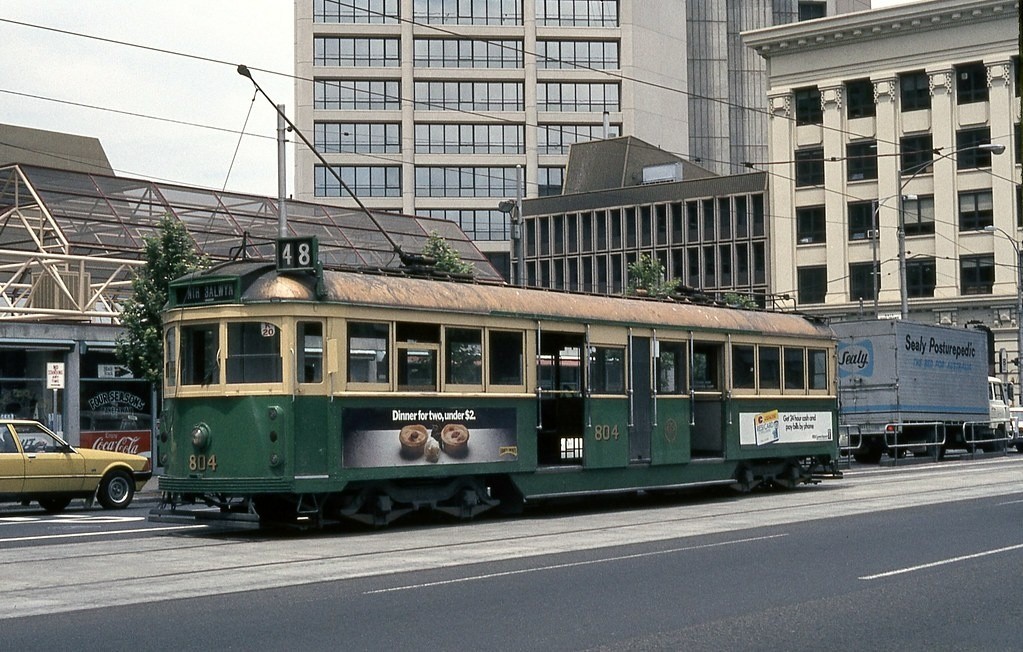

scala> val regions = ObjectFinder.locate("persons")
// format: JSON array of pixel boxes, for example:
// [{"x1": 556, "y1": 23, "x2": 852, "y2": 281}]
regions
[{"x1": 3, "y1": 431, "x2": 47, "y2": 453}]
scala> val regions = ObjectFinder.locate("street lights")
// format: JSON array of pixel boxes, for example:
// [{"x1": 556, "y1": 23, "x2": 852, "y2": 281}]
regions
[
  {"x1": 984, "y1": 225, "x2": 1023, "y2": 408},
  {"x1": 896, "y1": 143, "x2": 1006, "y2": 326}
]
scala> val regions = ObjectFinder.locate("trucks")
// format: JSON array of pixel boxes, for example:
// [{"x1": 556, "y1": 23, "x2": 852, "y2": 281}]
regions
[{"x1": 829, "y1": 318, "x2": 1016, "y2": 464}]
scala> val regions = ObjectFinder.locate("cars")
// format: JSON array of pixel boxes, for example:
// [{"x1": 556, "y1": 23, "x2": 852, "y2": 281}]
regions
[
  {"x1": 0, "y1": 418, "x2": 154, "y2": 513},
  {"x1": 1006, "y1": 406, "x2": 1023, "y2": 455}
]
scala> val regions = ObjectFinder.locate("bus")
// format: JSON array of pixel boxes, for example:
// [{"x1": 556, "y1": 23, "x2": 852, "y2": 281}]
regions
[{"x1": 154, "y1": 63, "x2": 844, "y2": 531}]
